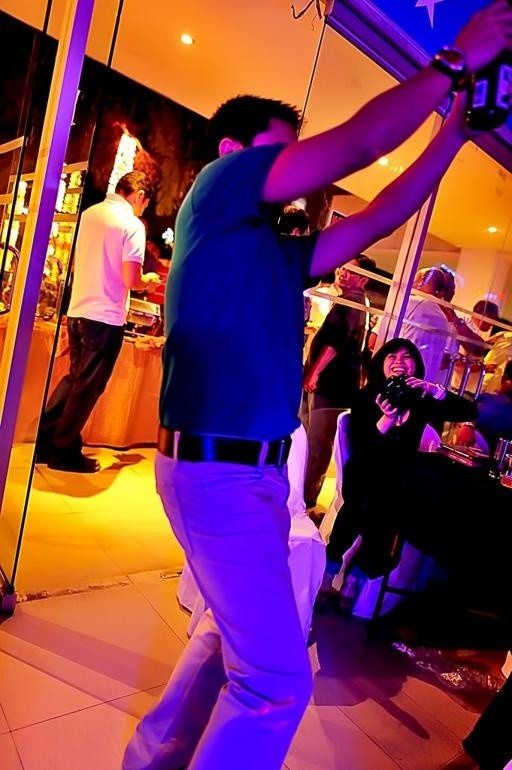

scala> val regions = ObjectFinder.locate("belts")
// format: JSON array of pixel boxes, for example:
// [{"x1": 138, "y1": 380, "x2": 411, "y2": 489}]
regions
[{"x1": 157, "y1": 426, "x2": 293, "y2": 468}]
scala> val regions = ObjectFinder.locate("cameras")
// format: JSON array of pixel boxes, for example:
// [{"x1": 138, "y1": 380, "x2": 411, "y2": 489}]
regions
[{"x1": 381, "y1": 375, "x2": 422, "y2": 416}]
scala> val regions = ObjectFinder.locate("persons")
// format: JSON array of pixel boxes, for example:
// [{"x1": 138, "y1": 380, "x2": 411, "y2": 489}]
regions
[
  {"x1": 302, "y1": 254, "x2": 377, "y2": 509},
  {"x1": 397, "y1": 268, "x2": 512, "y2": 402},
  {"x1": 448, "y1": 678, "x2": 512, "y2": 770},
  {"x1": 121, "y1": 0, "x2": 512, "y2": 770},
  {"x1": 35, "y1": 170, "x2": 161, "y2": 472},
  {"x1": 280, "y1": 205, "x2": 310, "y2": 237},
  {"x1": 315, "y1": 336, "x2": 479, "y2": 604}
]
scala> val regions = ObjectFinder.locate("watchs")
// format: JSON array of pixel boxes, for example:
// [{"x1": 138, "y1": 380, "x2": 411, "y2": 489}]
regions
[{"x1": 431, "y1": 43, "x2": 469, "y2": 92}]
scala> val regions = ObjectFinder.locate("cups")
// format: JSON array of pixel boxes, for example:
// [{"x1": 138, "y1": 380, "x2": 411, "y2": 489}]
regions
[{"x1": 489, "y1": 438, "x2": 512, "y2": 480}]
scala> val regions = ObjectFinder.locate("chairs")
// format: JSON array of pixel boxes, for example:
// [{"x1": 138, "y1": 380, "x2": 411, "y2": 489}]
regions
[
  {"x1": 320, "y1": 407, "x2": 492, "y2": 643},
  {"x1": 175, "y1": 418, "x2": 328, "y2": 645}
]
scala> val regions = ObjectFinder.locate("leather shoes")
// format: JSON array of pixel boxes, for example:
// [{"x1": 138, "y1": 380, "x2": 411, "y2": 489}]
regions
[{"x1": 31, "y1": 445, "x2": 102, "y2": 474}]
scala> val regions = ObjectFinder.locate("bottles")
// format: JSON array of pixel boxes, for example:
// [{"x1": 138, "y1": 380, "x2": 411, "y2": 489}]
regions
[{"x1": 465, "y1": 51, "x2": 512, "y2": 131}]
